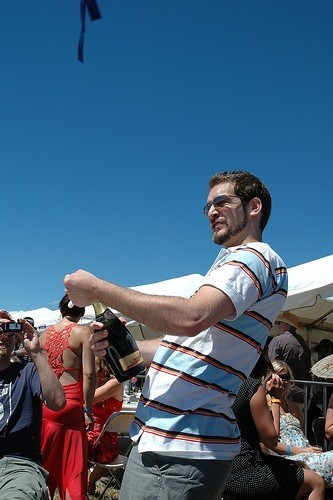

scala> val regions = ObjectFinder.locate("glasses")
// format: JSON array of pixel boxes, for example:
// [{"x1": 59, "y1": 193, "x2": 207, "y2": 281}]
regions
[
  {"x1": 279, "y1": 374, "x2": 291, "y2": 381},
  {"x1": 202, "y1": 194, "x2": 239, "y2": 215}
]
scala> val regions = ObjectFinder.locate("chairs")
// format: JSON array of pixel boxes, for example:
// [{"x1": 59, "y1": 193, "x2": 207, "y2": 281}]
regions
[{"x1": 88, "y1": 410, "x2": 137, "y2": 500}]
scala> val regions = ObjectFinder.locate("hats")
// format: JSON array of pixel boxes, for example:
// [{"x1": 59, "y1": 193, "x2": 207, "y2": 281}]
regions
[{"x1": 275, "y1": 312, "x2": 305, "y2": 332}]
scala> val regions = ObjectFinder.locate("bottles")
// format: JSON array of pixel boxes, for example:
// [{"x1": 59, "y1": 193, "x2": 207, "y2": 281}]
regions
[{"x1": 93, "y1": 302, "x2": 147, "y2": 382}]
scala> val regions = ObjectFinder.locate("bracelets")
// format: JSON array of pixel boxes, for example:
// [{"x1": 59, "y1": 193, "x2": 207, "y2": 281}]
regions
[
  {"x1": 83, "y1": 407, "x2": 93, "y2": 413},
  {"x1": 286, "y1": 445, "x2": 291, "y2": 456},
  {"x1": 269, "y1": 399, "x2": 282, "y2": 404}
]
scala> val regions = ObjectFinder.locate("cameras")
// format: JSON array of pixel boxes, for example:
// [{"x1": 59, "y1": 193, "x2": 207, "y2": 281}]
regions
[{"x1": 4, "y1": 323, "x2": 23, "y2": 332}]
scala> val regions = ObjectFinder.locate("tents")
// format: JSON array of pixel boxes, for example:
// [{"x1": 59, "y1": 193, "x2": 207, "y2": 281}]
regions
[{"x1": 0, "y1": 254, "x2": 333, "y2": 354}]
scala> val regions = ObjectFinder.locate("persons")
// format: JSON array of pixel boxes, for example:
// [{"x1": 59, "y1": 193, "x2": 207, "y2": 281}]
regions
[
  {"x1": 63, "y1": 170, "x2": 288, "y2": 500},
  {"x1": 38, "y1": 294, "x2": 95, "y2": 500},
  {"x1": 311, "y1": 339, "x2": 333, "y2": 441},
  {"x1": 0, "y1": 310, "x2": 67, "y2": 500},
  {"x1": 223, "y1": 348, "x2": 324, "y2": 500},
  {"x1": 85, "y1": 356, "x2": 125, "y2": 494},
  {"x1": 267, "y1": 311, "x2": 311, "y2": 434},
  {"x1": 260, "y1": 360, "x2": 333, "y2": 488}
]
